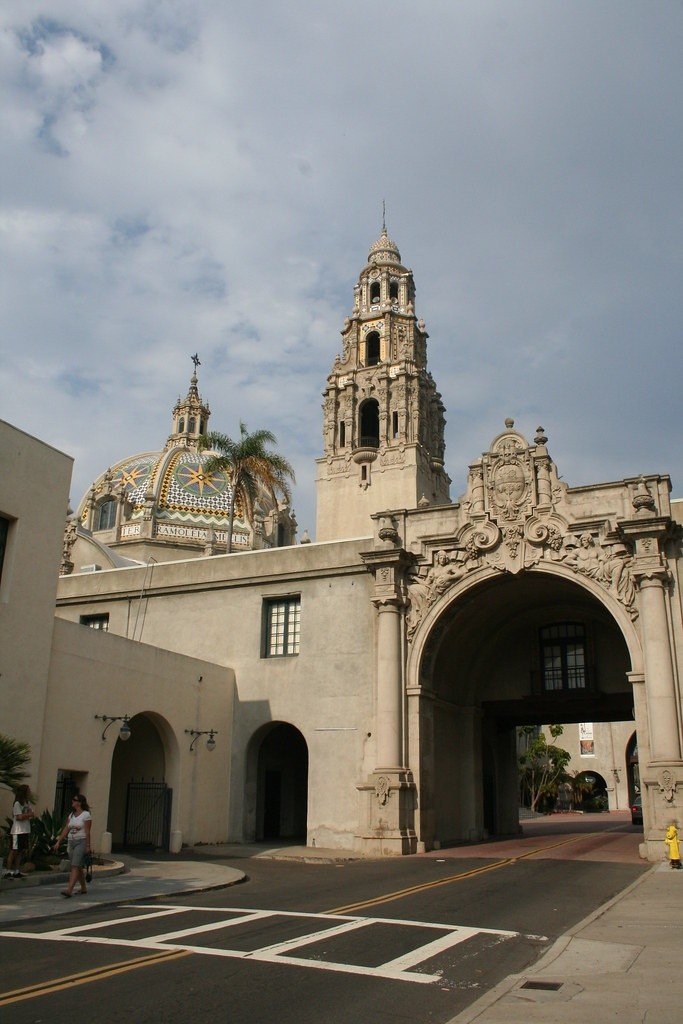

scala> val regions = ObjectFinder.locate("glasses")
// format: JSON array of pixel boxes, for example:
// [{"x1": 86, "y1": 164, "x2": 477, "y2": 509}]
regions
[{"x1": 73, "y1": 799, "x2": 78, "y2": 801}]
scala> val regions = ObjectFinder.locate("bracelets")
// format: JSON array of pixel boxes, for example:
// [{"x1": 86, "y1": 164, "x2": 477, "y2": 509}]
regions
[{"x1": 86, "y1": 844, "x2": 90, "y2": 847}]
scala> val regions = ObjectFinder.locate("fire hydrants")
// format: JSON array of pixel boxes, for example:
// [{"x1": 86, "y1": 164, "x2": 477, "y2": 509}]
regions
[{"x1": 664, "y1": 827, "x2": 681, "y2": 868}]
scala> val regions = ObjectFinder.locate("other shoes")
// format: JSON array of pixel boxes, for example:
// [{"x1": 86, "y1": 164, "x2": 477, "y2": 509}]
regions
[
  {"x1": 60, "y1": 890, "x2": 72, "y2": 897},
  {"x1": 15, "y1": 873, "x2": 25, "y2": 877},
  {"x1": 75, "y1": 889, "x2": 87, "y2": 894},
  {"x1": 4, "y1": 873, "x2": 13, "y2": 879}
]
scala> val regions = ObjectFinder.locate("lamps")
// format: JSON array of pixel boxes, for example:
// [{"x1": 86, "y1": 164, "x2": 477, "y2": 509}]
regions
[
  {"x1": 95, "y1": 714, "x2": 132, "y2": 741},
  {"x1": 613, "y1": 769, "x2": 618, "y2": 777},
  {"x1": 185, "y1": 729, "x2": 218, "y2": 751}
]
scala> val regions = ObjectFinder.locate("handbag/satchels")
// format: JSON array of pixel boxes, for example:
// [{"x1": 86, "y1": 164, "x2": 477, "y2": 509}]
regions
[{"x1": 86, "y1": 854, "x2": 92, "y2": 882}]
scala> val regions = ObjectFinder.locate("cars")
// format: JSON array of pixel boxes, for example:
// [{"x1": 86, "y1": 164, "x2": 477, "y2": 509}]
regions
[{"x1": 632, "y1": 794, "x2": 643, "y2": 825}]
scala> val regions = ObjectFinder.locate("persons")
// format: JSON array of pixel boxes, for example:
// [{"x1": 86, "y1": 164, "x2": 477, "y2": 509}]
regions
[
  {"x1": 405, "y1": 551, "x2": 463, "y2": 635},
  {"x1": 54, "y1": 794, "x2": 92, "y2": 897},
  {"x1": 4, "y1": 784, "x2": 35, "y2": 880},
  {"x1": 563, "y1": 533, "x2": 630, "y2": 606}
]
